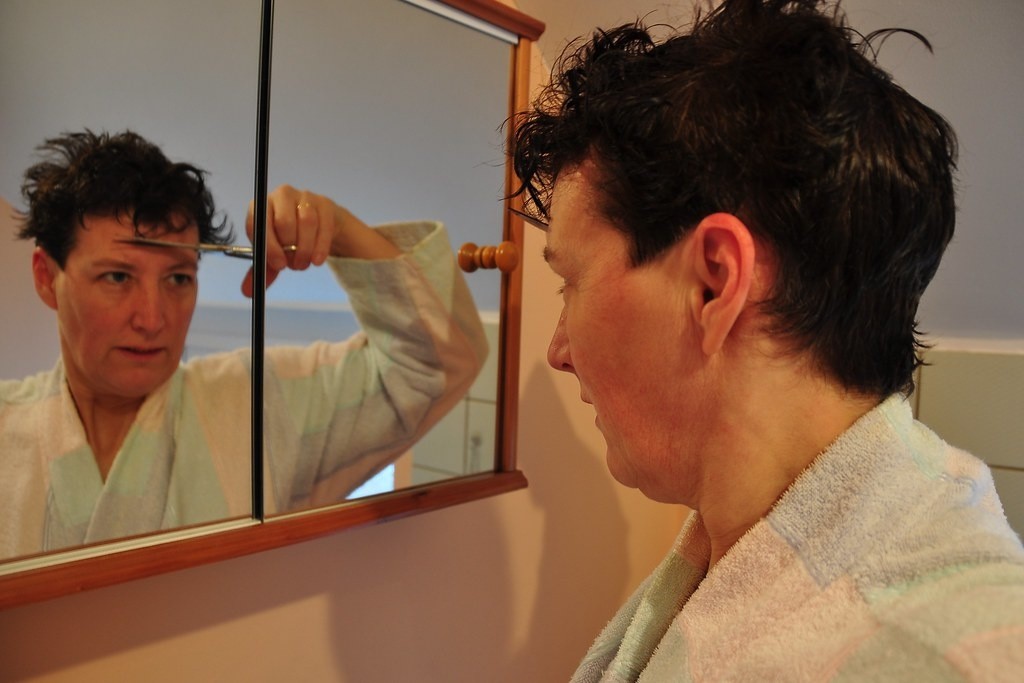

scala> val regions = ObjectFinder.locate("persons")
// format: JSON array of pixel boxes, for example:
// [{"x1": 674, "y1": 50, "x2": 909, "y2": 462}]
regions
[
  {"x1": 0, "y1": 125, "x2": 490, "y2": 560},
  {"x1": 498, "y1": 0, "x2": 1024, "y2": 682}
]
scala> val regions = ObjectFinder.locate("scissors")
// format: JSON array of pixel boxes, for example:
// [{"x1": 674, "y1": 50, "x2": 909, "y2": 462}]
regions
[
  {"x1": 113, "y1": 232, "x2": 299, "y2": 275},
  {"x1": 502, "y1": 200, "x2": 549, "y2": 234}
]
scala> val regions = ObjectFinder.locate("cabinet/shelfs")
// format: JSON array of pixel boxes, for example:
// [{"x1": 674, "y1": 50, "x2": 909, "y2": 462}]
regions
[{"x1": 0, "y1": 0, "x2": 547, "y2": 613}]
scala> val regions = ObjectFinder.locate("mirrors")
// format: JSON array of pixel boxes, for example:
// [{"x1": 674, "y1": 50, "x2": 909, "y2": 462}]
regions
[{"x1": 0, "y1": 0, "x2": 521, "y2": 560}]
